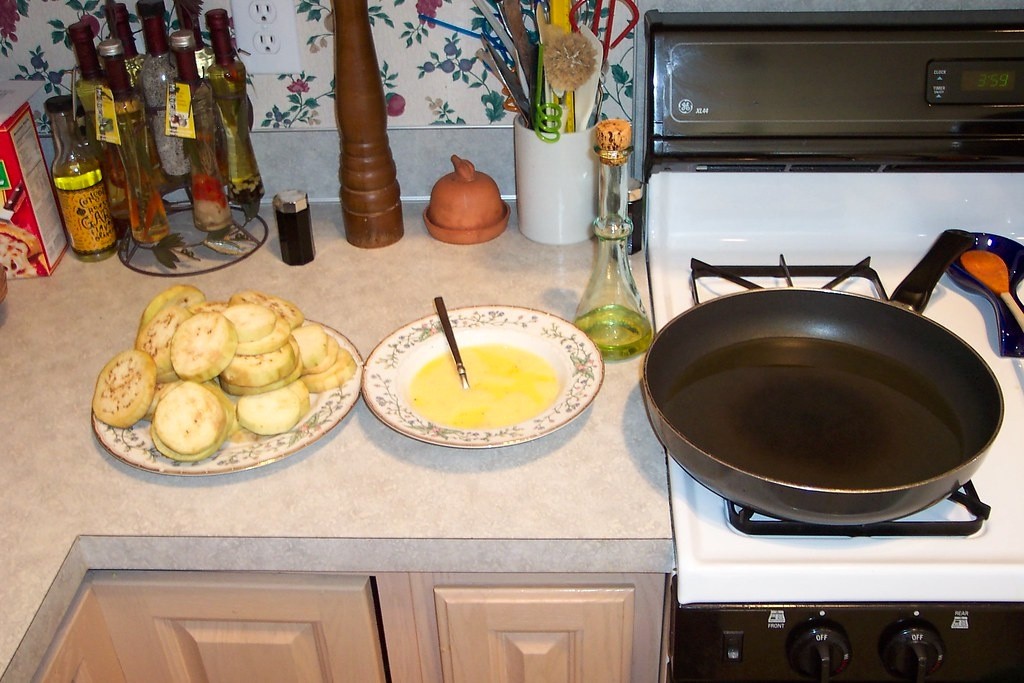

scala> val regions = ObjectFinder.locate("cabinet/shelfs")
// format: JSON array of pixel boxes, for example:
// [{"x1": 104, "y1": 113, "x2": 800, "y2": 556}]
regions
[{"x1": 31, "y1": 570, "x2": 669, "y2": 683}]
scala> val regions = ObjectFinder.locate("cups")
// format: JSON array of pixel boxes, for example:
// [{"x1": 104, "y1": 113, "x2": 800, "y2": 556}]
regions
[{"x1": 515, "y1": 115, "x2": 602, "y2": 246}]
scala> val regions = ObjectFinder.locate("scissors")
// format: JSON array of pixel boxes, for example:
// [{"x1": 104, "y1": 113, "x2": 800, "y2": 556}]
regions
[
  {"x1": 481, "y1": 33, "x2": 534, "y2": 130},
  {"x1": 569, "y1": 0, "x2": 639, "y2": 124}
]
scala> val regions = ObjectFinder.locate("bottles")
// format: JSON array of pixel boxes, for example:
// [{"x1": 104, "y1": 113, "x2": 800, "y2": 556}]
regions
[
  {"x1": 274, "y1": 189, "x2": 318, "y2": 264},
  {"x1": 43, "y1": 0, "x2": 265, "y2": 262},
  {"x1": 627, "y1": 178, "x2": 642, "y2": 254},
  {"x1": 575, "y1": 119, "x2": 655, "y2": 359}
]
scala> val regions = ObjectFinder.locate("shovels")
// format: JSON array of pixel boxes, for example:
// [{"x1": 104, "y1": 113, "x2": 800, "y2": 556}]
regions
[{"x1": 961, "y1": 251, "x2": 1024, "y2": 333}]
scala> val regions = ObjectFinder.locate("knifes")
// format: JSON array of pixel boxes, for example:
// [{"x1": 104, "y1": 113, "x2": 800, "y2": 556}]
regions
[{"x1": 483, "y1": 35, "x2": 530, "y2": 122}]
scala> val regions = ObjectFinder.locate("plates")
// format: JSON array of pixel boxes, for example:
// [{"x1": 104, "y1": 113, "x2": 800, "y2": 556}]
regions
[
  {"x1": 359, "y1": 306, "x2": 606, "y2": 449},
  {"x1": 92, "y1": 317, "x2": 364, "y2": 481}
]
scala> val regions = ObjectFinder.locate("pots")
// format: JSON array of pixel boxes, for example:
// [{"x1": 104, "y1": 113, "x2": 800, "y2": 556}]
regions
[{"x1": 642, "y1": 229, "x2": 1006, "y2": 524}]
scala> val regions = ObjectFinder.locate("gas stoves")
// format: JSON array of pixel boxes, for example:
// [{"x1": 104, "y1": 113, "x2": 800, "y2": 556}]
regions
[{"x1": 642, "y1": 9, "x2": 1023, "y2": 605}]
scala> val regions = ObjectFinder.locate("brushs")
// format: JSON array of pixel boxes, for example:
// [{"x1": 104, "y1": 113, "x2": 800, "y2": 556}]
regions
[{"x1": 542, "y1": 34, "x2": 598, "y2": 133}]
